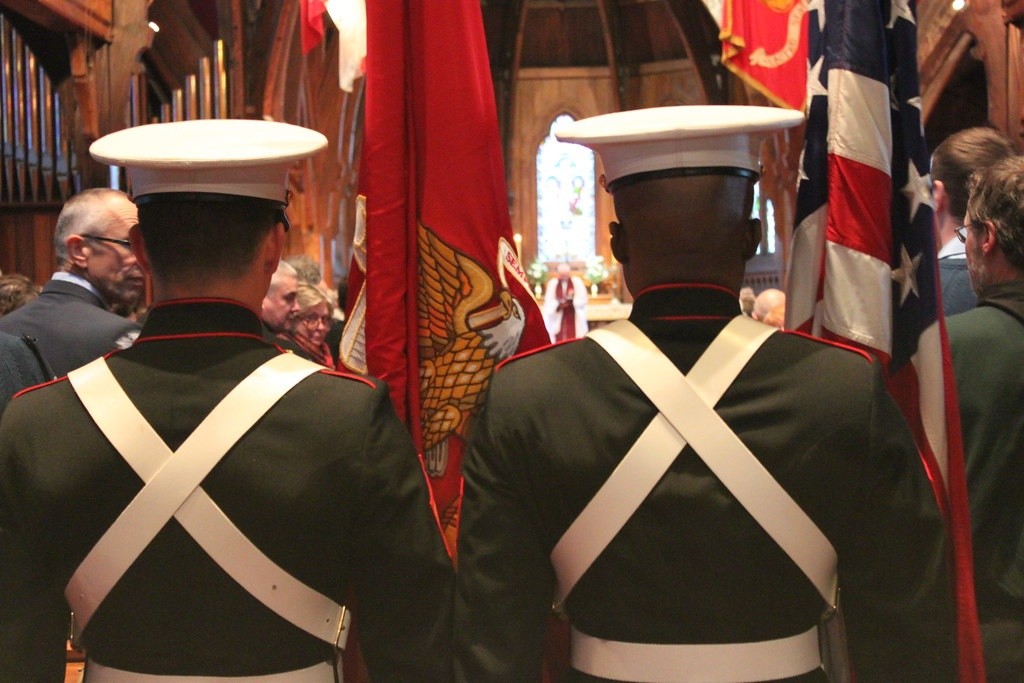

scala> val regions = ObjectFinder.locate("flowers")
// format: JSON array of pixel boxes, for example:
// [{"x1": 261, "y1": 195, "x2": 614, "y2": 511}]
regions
[
  {"x1": 581, "y1": 254, "x2": 609, "y2": 285},
  {"x1": 524, "y1": 256, "x2": 550, "y2": 285}
]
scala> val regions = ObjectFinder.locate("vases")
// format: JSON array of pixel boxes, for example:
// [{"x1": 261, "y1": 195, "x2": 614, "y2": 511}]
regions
[
  {"x1": 590, "y1": 283, "x2": 598, "y2": 298},
  {"x1": 534, "y1": 281, "x2": 542, "y2": 299}
]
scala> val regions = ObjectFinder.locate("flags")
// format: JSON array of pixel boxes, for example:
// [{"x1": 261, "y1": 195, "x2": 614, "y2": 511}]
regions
[
  {"x1": 718, "y1": 1, "x2": 986, "y2": 683},
  {"x1": 300, "y1": 0, "x2": 552, "y2": 558}
]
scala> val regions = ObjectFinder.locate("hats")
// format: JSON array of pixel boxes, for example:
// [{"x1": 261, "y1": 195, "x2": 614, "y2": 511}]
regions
[
  {"x1": 88, "y1": 119, "x2": 329, "y2": 231},
  {"x1": 554, "y1": 106, "x2": 806, "y2": 194}
]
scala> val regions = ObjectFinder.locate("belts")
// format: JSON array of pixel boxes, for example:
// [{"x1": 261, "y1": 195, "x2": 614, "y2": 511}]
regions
[
  {"x1": 85, "y1": 659, "x2": 335, "y2": 683},
  {"x1": 569, "y1": 626, "x2": 822, "y2": 683}
]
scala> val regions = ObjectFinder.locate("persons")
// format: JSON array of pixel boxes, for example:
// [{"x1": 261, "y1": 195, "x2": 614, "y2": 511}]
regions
[
  {"x1": 0, "y1": 117, "x2": 458, "y2": 683},
  {"x1": 0, "y1": 186, "x2": 349, "y2": 372},
  {"x1": 543, "y1": 262, "x2": 589, "y2": 344},
  {"x1": 944, "y1": 153, "x2": 1024, "y2": 683},
  {"x1": 741, "y1": 287, "x2": 757, "y2": 316},
  {"x1": 930, "y1": 125, "x2": 1015, "y2": 314},
  {"x1": 752, "y1": 289, "x2": 787, "y2": 329},
  {"x1": 452, "y1": 105, "x2": 944, "y2": 683}
]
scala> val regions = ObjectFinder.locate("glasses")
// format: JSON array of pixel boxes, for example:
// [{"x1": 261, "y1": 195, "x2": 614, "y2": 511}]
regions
[
  {"x1": 954, "y1": 223, "x2": 975, "y2": 243},
  {"x1": 297, "y1": 315, "x2": 333, "y2": 331},
  {"x1": 81, "y1": 234, "x2": 131, "y2": 248}
]
